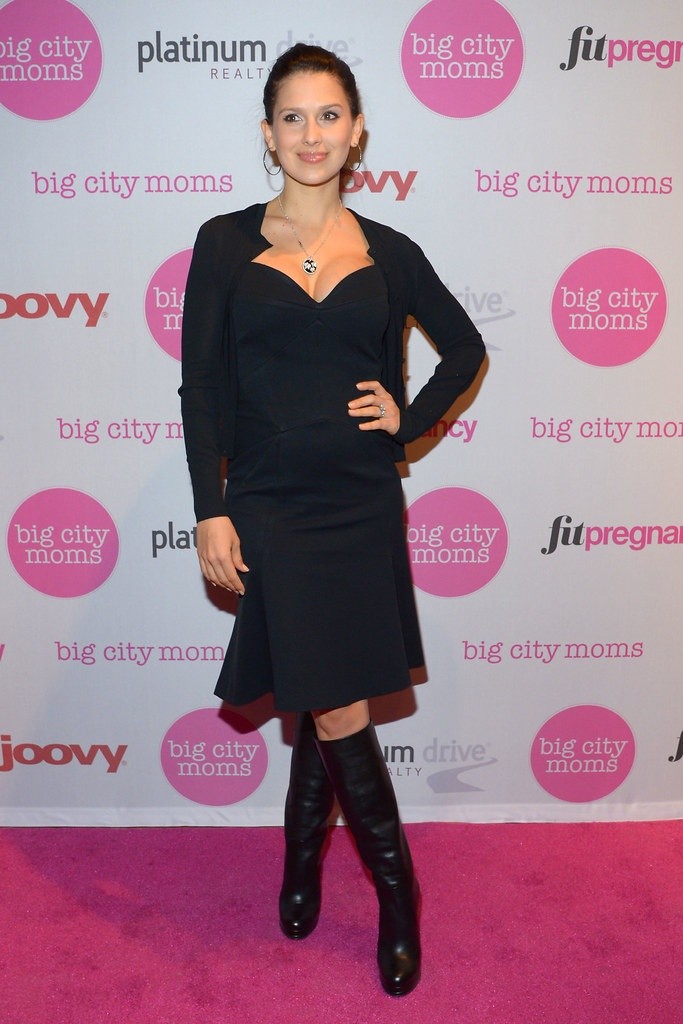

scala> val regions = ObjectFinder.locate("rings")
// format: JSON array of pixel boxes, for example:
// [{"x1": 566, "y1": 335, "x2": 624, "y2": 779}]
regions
[{"x1": 379, "y1": 403, "x2": 386, "y2": 417}]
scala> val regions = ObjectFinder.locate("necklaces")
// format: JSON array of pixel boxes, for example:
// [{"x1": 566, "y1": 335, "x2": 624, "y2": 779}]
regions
[{"x1": 277, "y1": 194, "x2": 343, "y2": 274}]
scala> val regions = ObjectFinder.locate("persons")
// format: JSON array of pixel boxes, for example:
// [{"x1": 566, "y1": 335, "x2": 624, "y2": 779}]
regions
[{"x1": 177, "y1": 42, "x2": 489, "y2": 997}]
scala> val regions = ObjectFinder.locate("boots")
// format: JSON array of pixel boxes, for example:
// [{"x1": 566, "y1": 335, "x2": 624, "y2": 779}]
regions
[
  {"x1": 314, "y1": 730, "x2": 418, "y2": 995},
  {"x1": 279, "y1": 711, "x2": 335, "y2": 939}
]
